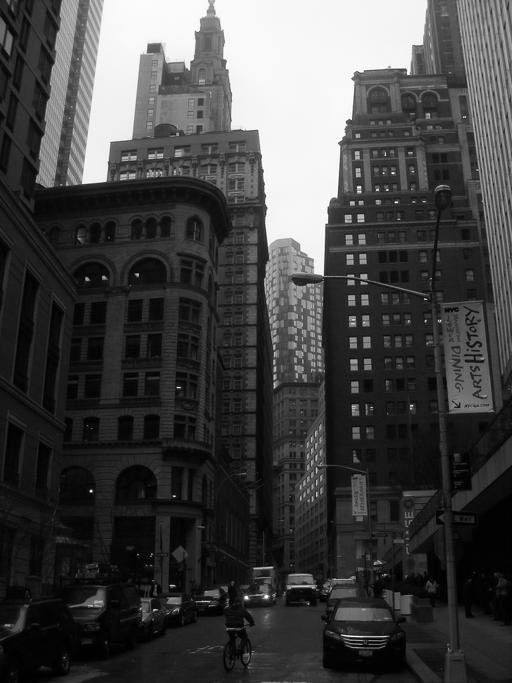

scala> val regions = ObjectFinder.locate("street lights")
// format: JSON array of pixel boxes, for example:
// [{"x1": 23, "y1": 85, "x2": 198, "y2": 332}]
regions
[
  {"x1": 291, "y1": 184, "x2": 470, "y2": 682},
  {"x1": 318, "y1": 464, "x2": 378, "y2": 592}
]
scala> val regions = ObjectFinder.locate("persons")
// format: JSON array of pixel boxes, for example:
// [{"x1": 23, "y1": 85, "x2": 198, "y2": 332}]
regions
[
  {"x1": 149, "y1": 578, "x2": 162, "y2": 598},
  {"x1": 461, "y1": 566, "x2": 512, "y2": 626},
  {"x1": 424, "y1": 576, "x2": 440, "y2": 608},
  {"x1": 23, "y1": 583, "x2": 34, "y2": 598},
  {"x1": 223, "y1": 595, "x2": 255, "y2": 660}
]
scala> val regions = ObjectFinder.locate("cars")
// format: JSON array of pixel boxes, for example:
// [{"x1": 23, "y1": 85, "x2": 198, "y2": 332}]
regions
[
  {"x1": 168, "y1": 595, "x2": 198, "y2": 625},
  {"x1": 196, "y1": 588, "x2": 229, "y2": 615},
  {"x1": 140, "y1": 598, "x2": 168, "y2": 638},
  {"x1": 320, "y1": 577, "x2": 365, "y2": 614},
  {"x1": 0, "y1": 600, "x2": 74, "y2": 682},
  {"x1": 320, "y1": 599, "x2": 407, "y2": 673},
  {"x1": 243, "y1": 583, "x2": 277, "y2": 607}
]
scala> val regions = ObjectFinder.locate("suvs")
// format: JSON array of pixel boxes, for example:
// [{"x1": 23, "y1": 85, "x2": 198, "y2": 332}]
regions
[
  {"x1": 286, "y1": 574, "x2": 317, "y2": 606},
  {"x1": 67, "y1": 581, "x2": 141, "y2": 660}
]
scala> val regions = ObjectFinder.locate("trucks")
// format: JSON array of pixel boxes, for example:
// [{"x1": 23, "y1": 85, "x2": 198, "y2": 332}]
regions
[{"x1": 252, "y1": 566, "x2": 277, "y2": 590}]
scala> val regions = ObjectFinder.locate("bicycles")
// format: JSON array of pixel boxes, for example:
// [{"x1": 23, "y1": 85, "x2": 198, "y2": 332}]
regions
[{"x1": 223, "y1": 624, "x2": 252, "y2": 671}]
